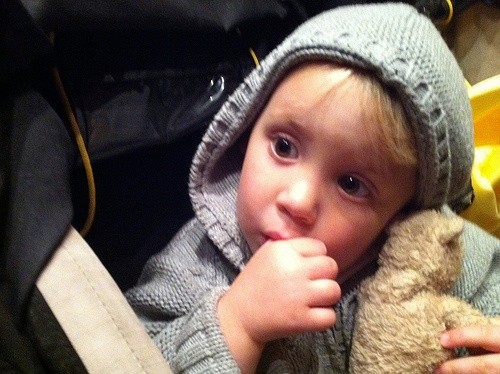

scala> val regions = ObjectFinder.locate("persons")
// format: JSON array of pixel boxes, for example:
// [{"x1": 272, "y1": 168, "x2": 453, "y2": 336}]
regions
[{"x1": 123, "y1": 2, "x2": 499, "y2": 373}]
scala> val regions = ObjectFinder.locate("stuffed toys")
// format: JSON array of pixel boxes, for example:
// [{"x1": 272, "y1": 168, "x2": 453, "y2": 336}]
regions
[{"x1": 344, "y1": 206, "x2": 488, "y2": 374}]
